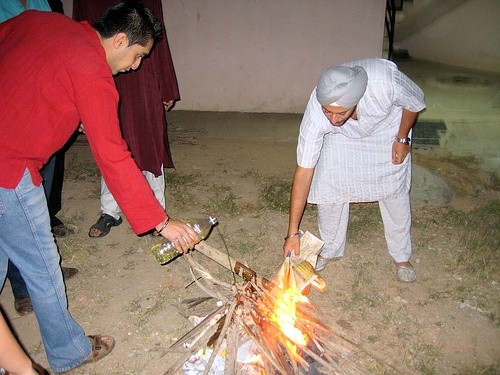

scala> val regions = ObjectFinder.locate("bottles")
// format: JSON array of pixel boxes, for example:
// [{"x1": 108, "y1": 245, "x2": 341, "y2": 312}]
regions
[{"x1": 149, "y1": 216, "x2": 218, "y2": 265}]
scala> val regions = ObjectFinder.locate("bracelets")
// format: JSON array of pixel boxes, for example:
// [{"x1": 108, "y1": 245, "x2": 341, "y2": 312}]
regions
[
  {"x1": 284, "y1": 233, "x2": 299, "y2": 240},
  {"x1": 158, "y1": 217, "x2": 171, "y2": 233}
]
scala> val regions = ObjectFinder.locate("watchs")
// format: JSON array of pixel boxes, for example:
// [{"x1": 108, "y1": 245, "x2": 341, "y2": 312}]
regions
[{"x1": 396, "y1": 137, "x2": 410, "y2": 145}]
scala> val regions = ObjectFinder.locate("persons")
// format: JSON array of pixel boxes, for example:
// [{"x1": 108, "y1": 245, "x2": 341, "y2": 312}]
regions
[
  {"x1": 7, "y1": 252, "x2": 78, "y2": 316},
  {"x1": 283, "y1": 58, "x2": 426, "y2": 283},
  {"x1": 1, "y1": 311, "x2": 45, "y2": 375},
  {"x1": 0, "y1": 2, "x2": 201, "y2": 373},
  {"x1": 0, "y1": 0, "x2": 75, "y2": 236},
  {"x1": 72, "y1": 0, "x2": 181, "y2": 238}
]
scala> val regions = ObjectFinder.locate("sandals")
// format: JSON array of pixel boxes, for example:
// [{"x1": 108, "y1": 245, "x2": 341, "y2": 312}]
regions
[
  {"x1": 397, "y1": 260, "x2": 417, "y2": 282},
  {"x1": 89, "y1": 213, "x2": 123, "y2": 238},
  {"x1": 87, "y1": 334, "x2": 115, "y2": 362},
  {"x1": 315, "y1": 257, "x2": 325, "y2": 272}
]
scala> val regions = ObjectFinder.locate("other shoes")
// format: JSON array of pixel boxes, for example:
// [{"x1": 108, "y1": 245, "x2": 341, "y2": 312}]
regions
[
  {"x1": 15, "y1": 298, "x2": 32, "y2": 315},
  {"x1": 53, "y1": 224, "x2": 73, "y2": 237},
  {"x1": 61, "y1": 267, "x2": 79, "y2": 279}
]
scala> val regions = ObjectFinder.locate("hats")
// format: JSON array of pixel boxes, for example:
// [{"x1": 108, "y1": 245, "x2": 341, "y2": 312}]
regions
[{"x1": 316, "y1": 65, "x2": 368, "y2": 109}]
